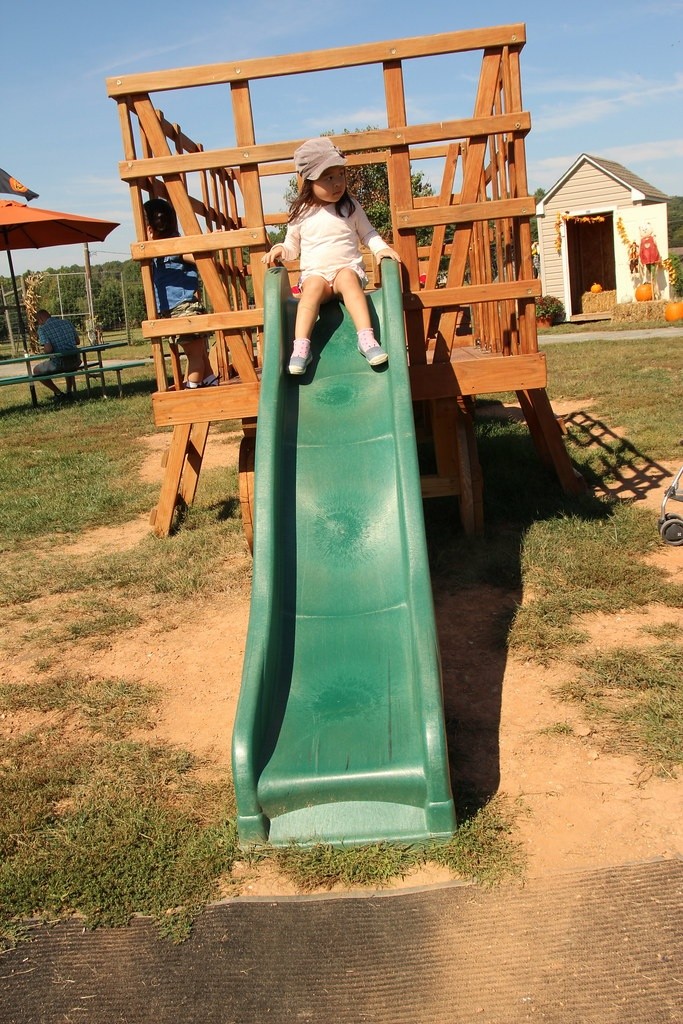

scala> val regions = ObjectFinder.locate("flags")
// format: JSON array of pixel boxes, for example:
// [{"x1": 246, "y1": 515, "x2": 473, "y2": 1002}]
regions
[{"x1": 0, "y1": 168, "x2": 40, "y2": 202}]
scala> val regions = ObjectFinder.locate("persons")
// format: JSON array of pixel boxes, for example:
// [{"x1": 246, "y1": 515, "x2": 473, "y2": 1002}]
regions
[
  {"x1": 32, "y1": 309, "x2": 81, "y2": 401},
  {"x1": 135, "y1": 199, "x2": 219, "y2": 391},
  {"x1": 259, "y1": 136, "x2": 404, "y2": 375}
]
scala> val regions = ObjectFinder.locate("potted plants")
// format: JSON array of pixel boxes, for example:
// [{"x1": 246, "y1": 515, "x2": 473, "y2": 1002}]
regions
[{"x1": 536, "y1": 314, "x2": 554, "y2": 329}]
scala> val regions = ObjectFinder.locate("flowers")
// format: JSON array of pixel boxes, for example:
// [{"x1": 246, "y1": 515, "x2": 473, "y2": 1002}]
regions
[{"x1": 536, "y1": 295, "x2": 565, "y2": 319}]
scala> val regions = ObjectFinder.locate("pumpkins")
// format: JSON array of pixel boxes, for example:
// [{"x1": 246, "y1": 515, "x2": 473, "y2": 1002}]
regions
[
  {"x1": 665, "y1": 301, "x2": 683, "y2": 322},
  {"x1": 635, "y1": 282, "x2": 652, "y2": 301},
  {"x1": 591, "y1": 282, "x2": 602, "y2": 293}
]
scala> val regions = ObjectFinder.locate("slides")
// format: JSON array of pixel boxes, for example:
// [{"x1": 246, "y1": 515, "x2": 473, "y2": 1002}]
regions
[{"x1": 232, "y1": 256, "x2": 456, "y2": 837}]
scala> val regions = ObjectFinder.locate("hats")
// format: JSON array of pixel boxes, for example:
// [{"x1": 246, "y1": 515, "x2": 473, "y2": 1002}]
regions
[{"x1": 294, "y1": 137, "x2": 348, "y2": 181}]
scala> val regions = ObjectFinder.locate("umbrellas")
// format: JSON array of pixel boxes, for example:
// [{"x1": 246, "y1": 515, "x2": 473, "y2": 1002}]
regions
[{"x1": 0, "y1": 195, "x2": 120, "y2": 257}]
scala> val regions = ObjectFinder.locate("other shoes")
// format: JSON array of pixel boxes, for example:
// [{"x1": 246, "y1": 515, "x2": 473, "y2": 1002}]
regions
[
  {"x1": 184, "y1": 372, "x2": 222, "y2": 390},
  {"x1": 358, "y1": 339, "x2": 388, "y2": 367},
  {"x1": 285, "y1": 348, "x2": 313, "y2": 375},
  {"x1": 67, "y1": 391, "x2": 74, "y2": 397},
  {"x1": 50, "y1": 392, "x2": 65, "y2": 401}
]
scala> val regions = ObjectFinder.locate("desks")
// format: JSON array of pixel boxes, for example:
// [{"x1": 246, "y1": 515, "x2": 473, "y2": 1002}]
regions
[{"x1": 0, "y1": 342, "x2": 128, "y2": 407}]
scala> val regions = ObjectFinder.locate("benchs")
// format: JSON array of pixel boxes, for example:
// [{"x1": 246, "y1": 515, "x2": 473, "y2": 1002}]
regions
[{"x1": 0, "y1": 361, "x2": 145, "y2": 385}]
